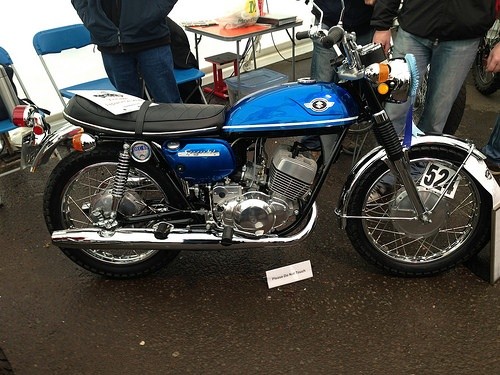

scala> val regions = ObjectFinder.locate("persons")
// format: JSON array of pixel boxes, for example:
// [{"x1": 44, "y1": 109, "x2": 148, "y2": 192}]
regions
[
  {"x1": 70, "y1": 0, "x2": 183, "y2": 105},
  {"x1": 305, "y1": 0, "x2": 500, "y2": 176}
]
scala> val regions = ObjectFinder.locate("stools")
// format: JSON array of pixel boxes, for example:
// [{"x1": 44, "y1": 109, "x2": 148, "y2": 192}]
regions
[{"x1": 205, "y1": 51, "x2": 244, "y2": 95}]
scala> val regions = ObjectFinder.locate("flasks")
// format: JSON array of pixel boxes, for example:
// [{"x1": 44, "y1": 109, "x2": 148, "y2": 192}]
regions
[{"x1": 0, "y1": 65, "x2": 20, "y2": 120}]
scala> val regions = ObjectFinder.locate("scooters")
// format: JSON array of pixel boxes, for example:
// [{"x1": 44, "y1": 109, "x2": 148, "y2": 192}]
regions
[{"x1": 473, "y1": 19, "x2": 500, "y2": 94}]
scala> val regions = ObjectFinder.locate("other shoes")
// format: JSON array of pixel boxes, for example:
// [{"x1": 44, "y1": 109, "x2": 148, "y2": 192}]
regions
[
  {"x1": 366, "y1": 181, "x2": 389, "y2": 202},
  {"x1": 485, "y1": 159, "x2": 500, "y2": 175},
  {"x1": 344, "y1": 132, "x2": 367, "y2": 154},
  {"x1": 302, "y1": 150, "x2": 321, "y2": 162}
]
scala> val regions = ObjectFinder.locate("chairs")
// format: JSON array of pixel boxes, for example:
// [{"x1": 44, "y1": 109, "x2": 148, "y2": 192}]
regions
[
  {"x1": 173, "y1": 65, "x2": 208, "y2": 104},
  {"x1": 0, "y1": 47, "x2": 63, "y2": 177},
  {"x1": 31, "y1": 21, "x2": 118, "y2": 113}
]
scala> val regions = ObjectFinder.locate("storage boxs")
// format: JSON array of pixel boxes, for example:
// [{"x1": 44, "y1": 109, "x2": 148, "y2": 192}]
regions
[{"x1": 224, "y1": 66, "x2": 289, "y2": 108}]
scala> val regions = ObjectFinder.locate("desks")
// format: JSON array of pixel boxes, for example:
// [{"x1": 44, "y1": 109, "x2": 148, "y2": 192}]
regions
[{"x1": 184, "y1": 17, "x2": 304, "y2": 100}]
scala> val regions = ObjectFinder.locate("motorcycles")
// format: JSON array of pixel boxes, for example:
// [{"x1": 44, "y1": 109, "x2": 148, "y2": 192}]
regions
[{"x1": 12, "y1": 1, "x2": 500, "y2": 281}]
